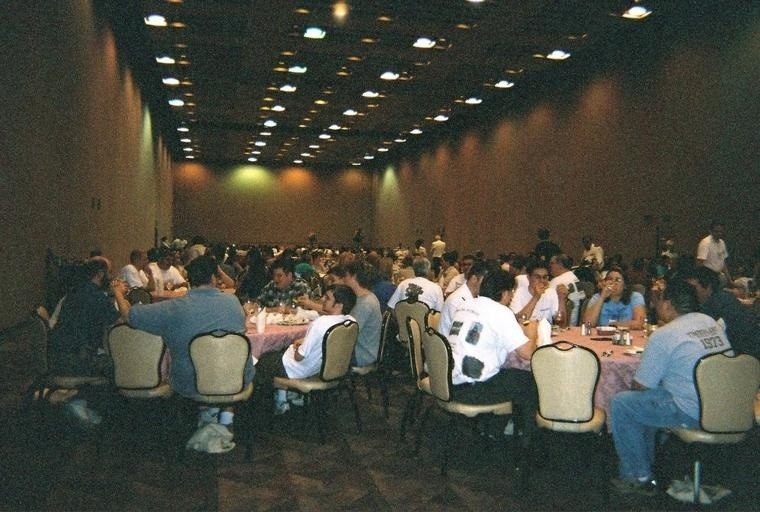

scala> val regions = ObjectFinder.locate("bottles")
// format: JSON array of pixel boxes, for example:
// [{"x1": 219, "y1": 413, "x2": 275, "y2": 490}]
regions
[
  {"x1": 555, "y1": 311, "x2": 657, "y2": 345},
  {"x1": 245, "y1": 290, "x2": 299, "y2": 325}
]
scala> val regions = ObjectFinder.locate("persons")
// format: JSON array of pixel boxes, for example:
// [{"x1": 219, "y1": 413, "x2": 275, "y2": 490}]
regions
[{"x1": 44, "y1": 219, "x2": 760, "y2": 502}]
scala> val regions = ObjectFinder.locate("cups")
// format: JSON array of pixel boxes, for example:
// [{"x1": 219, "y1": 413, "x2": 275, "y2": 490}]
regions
[{"x1": 601, "y1": 282, "x2": 615, "y2": 297}]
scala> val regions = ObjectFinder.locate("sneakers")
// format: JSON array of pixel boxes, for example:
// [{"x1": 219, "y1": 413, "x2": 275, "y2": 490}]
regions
[
  {"x1": 608, "y1": 476, "x2": 657, "y2": 497},
  {"x1": 292, "y1": 396, "x2": 304, "y2": 407},
  {"x1": 274, "y1": 403, "x2": 291, "y2": 415}
]
